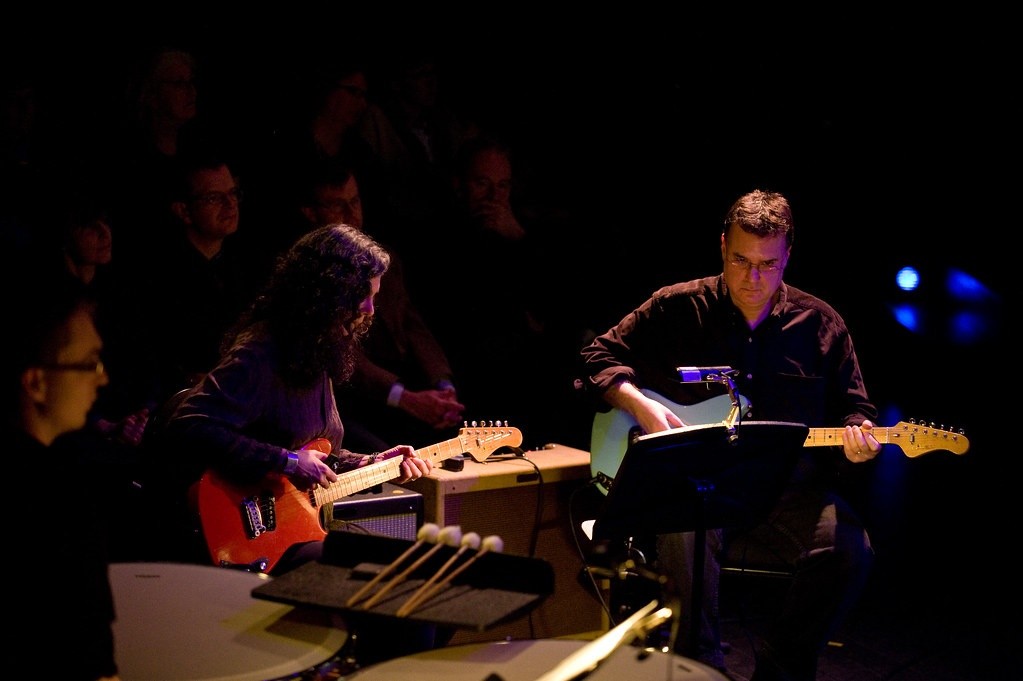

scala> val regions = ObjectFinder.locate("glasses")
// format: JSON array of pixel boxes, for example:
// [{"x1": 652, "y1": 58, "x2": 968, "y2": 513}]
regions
[
  {"x1": 726, "y1": 243, "x2": 786, "y2": 272},
  {"x1": 319, "y1": 195, "x2": 359, "y2": 212},
  {"x1": 196, "y1": 186, "x2": 242, "y2": 204},
  {"x1": 36, "y1": 359, "x2": 104, "y2": 377}
]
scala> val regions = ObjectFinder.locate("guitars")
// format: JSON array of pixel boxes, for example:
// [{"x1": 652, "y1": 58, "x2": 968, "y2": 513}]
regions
[
  {"x1": 594, "y1": 389, "x2": 968, "y2": 498},
  {"x1": 200, "y1": 419, "x2": 523, "y2": 580}
]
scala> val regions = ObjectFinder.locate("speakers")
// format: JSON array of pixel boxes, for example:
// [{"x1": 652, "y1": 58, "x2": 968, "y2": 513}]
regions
[
  {"x1": 334, "y1": 482, "x2": 424, "y2": 543},
  {"x1": 401, "y1": 441, "x2": 613, "y2": 647}
]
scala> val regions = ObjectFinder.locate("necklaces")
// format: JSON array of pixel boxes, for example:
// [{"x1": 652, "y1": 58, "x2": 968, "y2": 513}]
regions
[{"x1": 855, "y1": 448, "x2": 862, "y2": 455}]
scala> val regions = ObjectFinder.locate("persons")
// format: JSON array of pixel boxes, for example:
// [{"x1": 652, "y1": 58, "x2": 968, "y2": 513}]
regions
[
  {"x1": 165, "y1": 219, "x2": 460, "y2": 678},
  {"x1": 573, "y1": 188, "x2": 880, "y2": 681},
  {"x1": 0, "y1": 39, "x2": 646, "y2": 568},
  {"x1": 1, "y1": 280, "x2": 166, "y2": 681}
]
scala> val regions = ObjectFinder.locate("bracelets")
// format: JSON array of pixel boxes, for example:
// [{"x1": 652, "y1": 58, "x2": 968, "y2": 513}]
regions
[
  {"x1": 368, "y1": 452, "x2": 381, "y2": 467},
  {"x1": 283, "y1": 449, "x2": 299, "y2": 473}
]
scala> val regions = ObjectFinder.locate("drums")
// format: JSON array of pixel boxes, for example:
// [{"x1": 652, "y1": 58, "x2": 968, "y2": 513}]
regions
[
  {"x1": 357, "y1": 639, "x2": 728, "y2": 681},
  {"x1": 112, "y1": 557, "x2": 346, "y2": 679}
]
